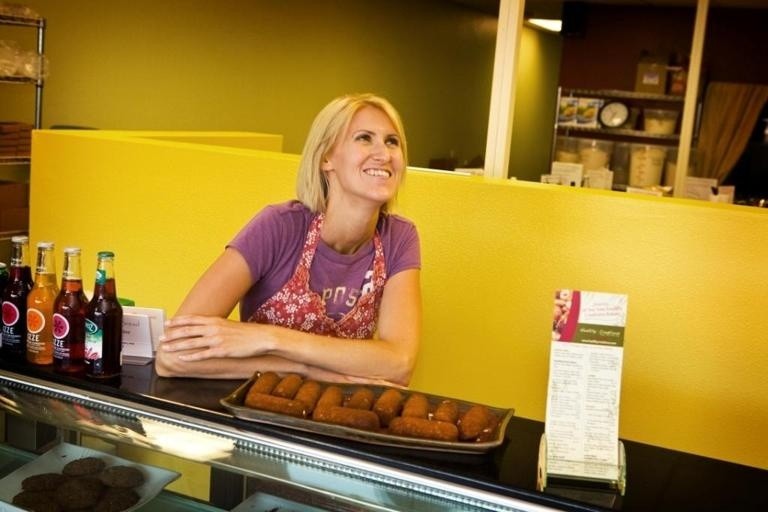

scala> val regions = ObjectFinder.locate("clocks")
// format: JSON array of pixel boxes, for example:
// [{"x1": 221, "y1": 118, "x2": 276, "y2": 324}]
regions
[{"x1": 594, "y1": 100, "x2": 629, "y2": 129}]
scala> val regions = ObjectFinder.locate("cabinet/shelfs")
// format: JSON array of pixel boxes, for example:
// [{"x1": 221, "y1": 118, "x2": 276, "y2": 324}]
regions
[
  {"x1": 548, "y1": 85, "x2": 682, "y2": 199},
  {"x1": 0, "y1": 350, "x2": 765, "y2": 512},
  {"x1": 1, "y1": 11, "x2": 47, "y2": 166}
]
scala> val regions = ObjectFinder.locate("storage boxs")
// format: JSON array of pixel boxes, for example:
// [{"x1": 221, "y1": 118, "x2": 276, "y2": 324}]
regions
[{"x1": 630, "y1": 60, "x2": 682, "y2": 96}]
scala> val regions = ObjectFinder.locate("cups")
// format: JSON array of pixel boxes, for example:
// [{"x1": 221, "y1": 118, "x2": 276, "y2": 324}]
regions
[{"x1": 554, "y1": 134, "x2": 694, "y2": 190}]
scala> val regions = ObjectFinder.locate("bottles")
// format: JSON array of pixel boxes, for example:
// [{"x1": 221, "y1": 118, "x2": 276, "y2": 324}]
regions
[
  {"x1": 26, "y1": 242, "x2": 58, "y2": 364},
  {"x1": 669, "y1": 46, "x2": 685, "y2": 96},
  {"x1": 0, "y1": 236, "x2": 34, "y2": 362},
  {"x1": 83, "y1": 253, "x2": 125, "y2": 379},
  {"x1": 52, "y1": 249, "x2": 87, "y2": 375}
]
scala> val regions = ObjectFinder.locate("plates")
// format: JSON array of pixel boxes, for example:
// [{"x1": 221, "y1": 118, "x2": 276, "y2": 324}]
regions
[{"x1": 0, "y1": 440, "x2": 184, "y2": 512}]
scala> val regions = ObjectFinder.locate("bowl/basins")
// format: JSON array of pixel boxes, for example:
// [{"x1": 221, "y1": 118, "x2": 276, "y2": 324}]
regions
[{"x1": 627, "y1": 107, "x2": 680, "y2": 134}]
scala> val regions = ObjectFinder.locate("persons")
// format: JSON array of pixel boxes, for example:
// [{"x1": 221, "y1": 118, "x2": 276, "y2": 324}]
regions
[{"x1": 154, "y1": 92, "x2": 421, "y2": 391}]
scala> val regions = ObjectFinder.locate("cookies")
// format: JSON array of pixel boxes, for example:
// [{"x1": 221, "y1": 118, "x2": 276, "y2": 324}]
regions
[{"x1": 11, "y1": 457, "x2": 144, "y2": 512}]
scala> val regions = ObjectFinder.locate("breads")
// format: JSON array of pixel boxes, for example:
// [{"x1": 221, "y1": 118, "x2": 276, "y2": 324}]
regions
[{"x1": 244, "y1": 371, "x2": 490, "y2": 443}]
